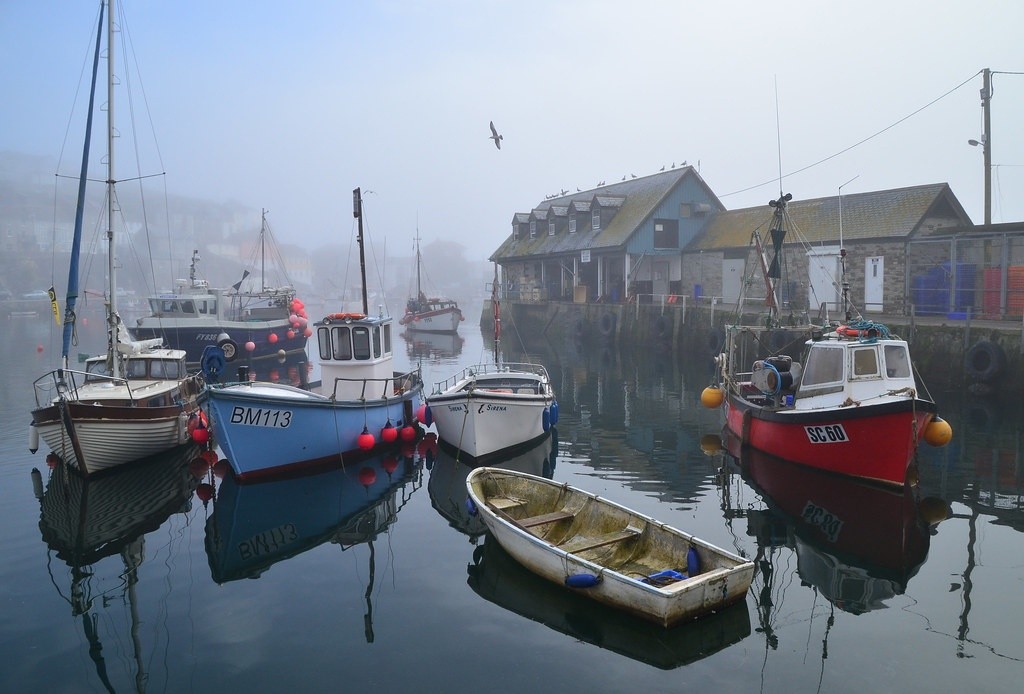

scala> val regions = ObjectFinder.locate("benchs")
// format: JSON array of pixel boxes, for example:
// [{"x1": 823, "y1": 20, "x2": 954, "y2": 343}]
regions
[
  {"x1": 515, "y1": 511, "x2": 574, "y2": 528},
  {"x1": 558, "y1": 528, "x2": 642, "y2": 553}
]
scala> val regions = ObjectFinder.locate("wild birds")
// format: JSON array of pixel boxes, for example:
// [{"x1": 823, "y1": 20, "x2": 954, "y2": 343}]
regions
[{"x1": 487, "y1": 120, "x2": 504, "y2": 151}]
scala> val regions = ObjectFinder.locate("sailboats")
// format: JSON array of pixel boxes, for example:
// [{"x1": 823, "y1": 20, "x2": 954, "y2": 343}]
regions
[
  {"x1": 37, "y1": 441, "x2": 206, "y2": 694},
  {"x1": 31, "y1": 0, "x2": 208, "y2": 482}
]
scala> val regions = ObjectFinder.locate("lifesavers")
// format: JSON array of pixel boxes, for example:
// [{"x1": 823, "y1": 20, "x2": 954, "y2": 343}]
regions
[
  {"x1": 704, "y1": 328, "x2": 722, "y2": 354},
  {"x1": 967, "y1": 342, "x2": 1007, "y2": 382},
  {"x1": 574, "y1": 319, "x2": 589, "y2": 338},
  {"x1": 598, "y1": 310, "x2": 615, "y2": 337},
  {"x1": 327, "y1": 312, "x2": 368, "y2": 319},
  {"x1": 836, "y1": 324, "x2": 881, "y2": 336},
  {"x1": 652, "y1": 315, "x2": 671, "y2": 338}
]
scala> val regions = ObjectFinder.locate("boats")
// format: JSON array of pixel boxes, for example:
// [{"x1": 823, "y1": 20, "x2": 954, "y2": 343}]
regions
[
  {"x1": 124, "y1": 206, "x2": 308, "y2": 373},
  {"x1": 423, "y1": 253, "x2": 559, "y2": 459},
  {"x1": 194, "y1": 187, "x2": 427, "y2": 486},
  {"x1": 203, "y1": 437, "x2": 428, "y2": 646},
  {"x1": 426, "y1": 437, "x2": 558, "y2": 541},
  {"x1": 721, "y1": 70, "x2": 934, "y2": 489},
  {"x1": 406, "y1": 329, "x2": 465, "y2": 357},
  {"x1": 465, "y1": 467, "x2": 755, "y2": 629},
  {"x1": 399, "y1": 226, "x2": 461, "y2": 331},
  {"x1": 724, "y1": 423, "x2": 933, "y2": 616},
  {"x1": 465, "y1": 530, "x2": 751, "y2": 671}
]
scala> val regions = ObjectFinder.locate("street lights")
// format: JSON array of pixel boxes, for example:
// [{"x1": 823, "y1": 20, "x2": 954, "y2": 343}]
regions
[{"x1": 967, "y1": 139, "x2": 992, "y2": 317}]
